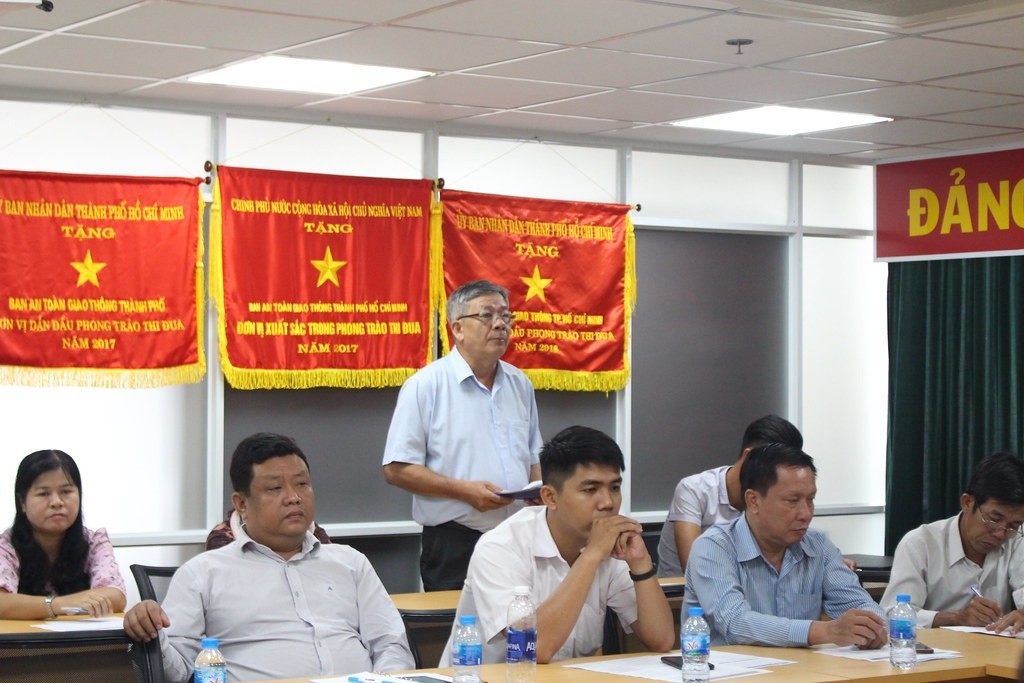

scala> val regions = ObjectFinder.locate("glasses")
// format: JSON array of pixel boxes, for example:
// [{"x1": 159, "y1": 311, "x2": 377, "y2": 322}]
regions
[
  {"x1": 974, "y1": 497, "x2": 1023, "y2": 539},
  {"x1": 457, "y1": 312, "x2": 517, "y2": 324}
]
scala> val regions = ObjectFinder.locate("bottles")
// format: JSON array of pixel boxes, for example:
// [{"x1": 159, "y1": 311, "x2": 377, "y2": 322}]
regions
[
  {"x1": 680, "y1": 608, "x2": 711, "y2": 683},
  {"x1": 194, "y1": 638, "x2": 227, "y2": 683},
  {"x1": 506, "y1": 586, "x2": 537, "y2": 683},
  {"x1": 889, "y1": 594, "x2": 917, "y2": 671},
  {"x1": 451, "y1": 615, "x2": 482, "y2": 683}
]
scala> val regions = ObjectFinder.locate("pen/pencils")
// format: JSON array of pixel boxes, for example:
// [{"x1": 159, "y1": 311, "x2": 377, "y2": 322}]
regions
[
  {"x1": 971, "y1": 586, "x2": 982, "y2": 596},
  {"x1": 347, "y1": 676, "x2": 392, "y2": 683},
  {"x1": 61, "y1": 606, "x2": 86, "y2": 613}
]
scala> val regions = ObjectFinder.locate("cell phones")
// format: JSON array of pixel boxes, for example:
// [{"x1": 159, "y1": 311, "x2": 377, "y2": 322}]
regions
[
  {"x1": 907, "y1": 641, "x2": 934, "y2": 653},
  {"x1": 661, "y1": 656, "x2": 715, "y2": 671}
]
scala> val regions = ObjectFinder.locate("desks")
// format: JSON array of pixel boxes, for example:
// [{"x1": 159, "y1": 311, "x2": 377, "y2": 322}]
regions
[{"x1": 0, "y1": 576, "x2": 1023, "y2": 682}]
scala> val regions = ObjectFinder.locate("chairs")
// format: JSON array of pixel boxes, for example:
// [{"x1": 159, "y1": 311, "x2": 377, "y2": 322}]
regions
[{"x1": 130, "y1": 565, "x2": 178, "y2": 683}]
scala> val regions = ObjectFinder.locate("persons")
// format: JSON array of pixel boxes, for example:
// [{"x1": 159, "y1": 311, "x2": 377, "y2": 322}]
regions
[
  {"x1": 123, "y1": 431, "x2": 415, "y2": 683},
  {"x1": 0, "y1": 449, "x2": 127, "y2": 619},
  {"x1": 206, "y1": 505, "x2": 332, "y2": 551},
  {"x1": 438, "y1": 425, "x2": 675, "y2": 668},
  {"x1": 381, "y1": 280, "x2": 544, "y2": 593},
  {"x1": 875, "y1": 451, "x2": 1024, "y2": 636},
  {"x1": 656, "y1": 414, "x2": 855, "y2": 577},
  {"x1": 679, "y1": 441, "x2": 889, "y2": 650}
]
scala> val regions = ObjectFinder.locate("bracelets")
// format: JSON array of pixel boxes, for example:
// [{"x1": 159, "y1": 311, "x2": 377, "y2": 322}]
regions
[
  {"x1": 45, "y1": 595, "x2": 57, "y2": 618},
  {"x1": 628, "y1": 564, "x2": 657, "y2": 581}
]
